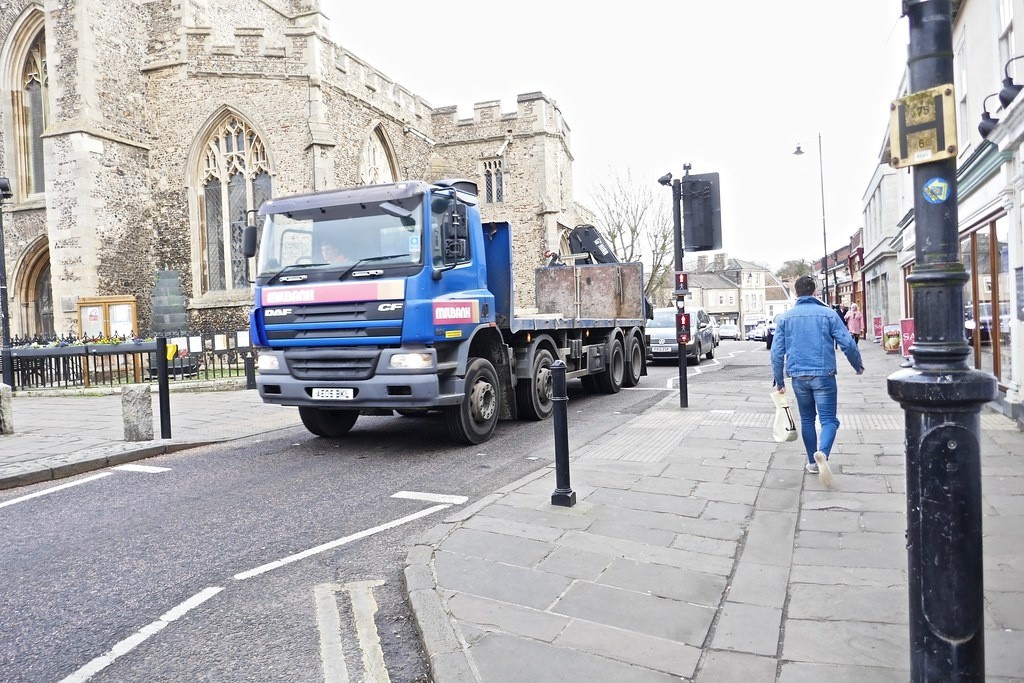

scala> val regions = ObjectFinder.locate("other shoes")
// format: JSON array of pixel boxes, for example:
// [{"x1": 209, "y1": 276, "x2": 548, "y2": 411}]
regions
[
  {"x1": 806, "y1": 462, "x2": 819, "y2": 474},
  {"x1": 813, "y1": 451, "x2": 834, "y2": 488}
]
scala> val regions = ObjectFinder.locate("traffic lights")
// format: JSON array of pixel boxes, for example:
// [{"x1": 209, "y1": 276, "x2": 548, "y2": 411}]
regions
[
  {"x1": 674, "y1": 271, "x2": 688, "y2": 295},
  {"x1": 675, "y1": 314, "x2": 691, "y2": 342}
]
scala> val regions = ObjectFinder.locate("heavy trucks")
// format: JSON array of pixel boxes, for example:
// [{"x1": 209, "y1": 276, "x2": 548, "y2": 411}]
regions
[{"x1": 243, "y1": 178, "x2": 644, "y2": 444}]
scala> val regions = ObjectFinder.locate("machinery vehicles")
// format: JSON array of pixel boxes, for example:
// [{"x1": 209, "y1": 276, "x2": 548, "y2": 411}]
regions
[{"x1": 544, "y1": 224, "x2": 655, "y2": 319}]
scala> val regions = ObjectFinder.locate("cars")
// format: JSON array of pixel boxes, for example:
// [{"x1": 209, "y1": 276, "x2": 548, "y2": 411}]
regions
[
  {"x1": 718, "y1": 324, "x2": 741, "y2": 341},
  {"x1": 745, "y1": 312, "x2": 782, "y2": 348},
  {"x1": 643, "y1": 306, "x2": 714, "y2": 364},
  {"x1": 964, "y1": 303, "x2": 1009, "y2": 347},
  {"x1": 709, "y1": 315, "x2": 720, "y2": 347}
]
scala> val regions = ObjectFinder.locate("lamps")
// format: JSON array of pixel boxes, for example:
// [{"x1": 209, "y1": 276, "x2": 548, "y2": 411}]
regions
[
  {"x1": 998, "y1": 55, "x2": 1024, "y2": 109},
  {"x1": 979, "y1": 93, "x2": 999, "y2": 140}
]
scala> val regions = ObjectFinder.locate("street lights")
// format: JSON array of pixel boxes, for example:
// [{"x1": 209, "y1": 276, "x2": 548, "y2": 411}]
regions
[
  {"x1": 0, "y1": 176, "x2": 16, "y2": 392},
  {"x1": 793, "y1": 137, "x2": 829, "y2": 306}
]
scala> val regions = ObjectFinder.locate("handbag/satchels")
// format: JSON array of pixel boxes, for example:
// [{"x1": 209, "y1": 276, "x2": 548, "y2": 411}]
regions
[{"x1": 769, "y1": 392, "x2": 798, "y2": 443}]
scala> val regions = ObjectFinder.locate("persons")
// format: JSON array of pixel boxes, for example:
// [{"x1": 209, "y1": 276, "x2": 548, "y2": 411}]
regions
[
  {"x1": 835, "y1": 305, "x2": 848, "y2": 349},
  {"x1": 770, "y1": 275, "x2": 865, "y2": 488},
  {"x1": 844, "y1": 303, "x2": 864, "y2": 346},
  {"x1": 320, "y1": 238, "x2": 349, "y2": 263}
]
